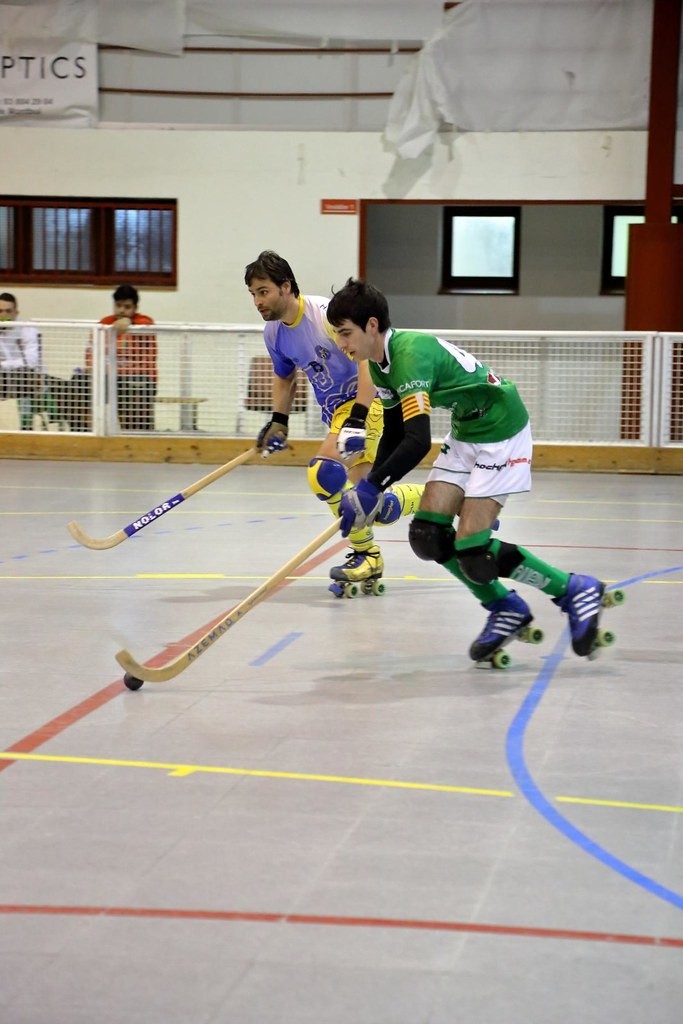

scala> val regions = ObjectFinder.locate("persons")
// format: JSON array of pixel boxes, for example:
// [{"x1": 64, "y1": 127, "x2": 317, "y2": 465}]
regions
[
  {"x1": 0, "y1": 293, "x2": 44, "y2": 399},
  {"x1": 245, "y1": 251, "x2": 426, "y2": 596},
  {"x1": 327, "y1": 283, "x2": 624, "y2": 669},
  {"x1": 86, "y1": 285, "x2": 158, "y2": 429}
]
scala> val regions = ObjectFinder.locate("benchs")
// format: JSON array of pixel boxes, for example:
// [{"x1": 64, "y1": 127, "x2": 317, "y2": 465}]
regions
[{"x1": 154, "y1": 397, "x2": 209, "y2": 429}]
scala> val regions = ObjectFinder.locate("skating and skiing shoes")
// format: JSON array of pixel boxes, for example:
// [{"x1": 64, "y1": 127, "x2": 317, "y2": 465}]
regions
[
  {"x1": 550, "y1": 572, "x2": 626, "y2": 662},
  {"x1": 468, "y1": 589, "x2": 544, "y2": 670},
  {"x1": 328, "y1": 545, "x2": 386, "y2": 598}
]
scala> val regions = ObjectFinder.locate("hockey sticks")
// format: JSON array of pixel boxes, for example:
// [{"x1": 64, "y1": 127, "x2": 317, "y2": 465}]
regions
[
  {"x1": 65, "y1": 431, "x2": 284, "y2": 551},
  {"x1": 114, "y1": 516, "x2": 345, "y2": 683}
]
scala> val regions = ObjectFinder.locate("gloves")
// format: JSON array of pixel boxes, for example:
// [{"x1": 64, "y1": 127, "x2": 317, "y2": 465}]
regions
[
  {"x1": 255, "y1": 412, "x2": 289, "y2": 459},
  {"x1": 335, "y1": 403, "x2": 369, "y2": 462},
  {"x1": 338, "y1": 478, "x2": 385, "y2": 538}
]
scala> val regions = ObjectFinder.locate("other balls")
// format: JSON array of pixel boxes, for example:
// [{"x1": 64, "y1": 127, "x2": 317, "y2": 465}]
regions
[{"x1": 123, "y1": 671, "x2": 144, "y2": 690}]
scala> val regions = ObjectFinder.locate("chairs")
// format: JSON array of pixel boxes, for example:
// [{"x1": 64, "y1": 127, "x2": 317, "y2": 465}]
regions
[{"x1": 239, "y1": 357, "x2": 308, "y2": 438}]
